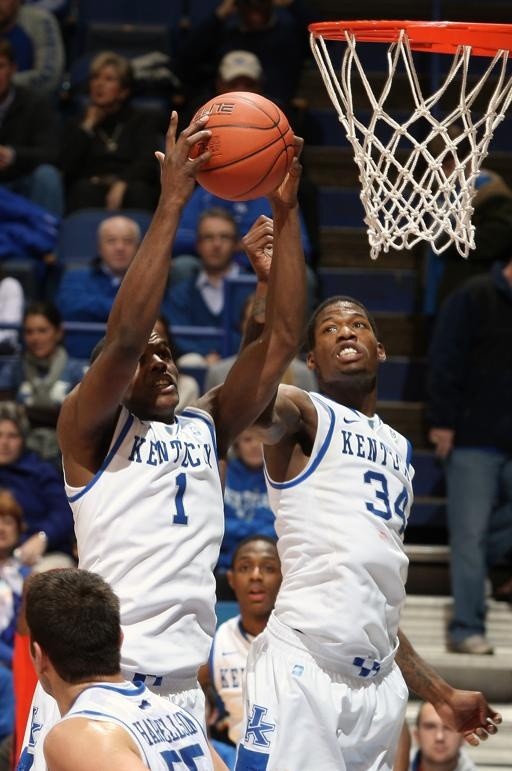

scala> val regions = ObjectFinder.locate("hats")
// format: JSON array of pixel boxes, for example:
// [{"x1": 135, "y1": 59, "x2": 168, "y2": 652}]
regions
[{"x1": 218, "y1": 49, "x2": 263, "y2": 82}]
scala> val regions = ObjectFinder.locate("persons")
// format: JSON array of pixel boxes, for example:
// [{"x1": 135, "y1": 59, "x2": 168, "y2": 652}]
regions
[
  {"x1": 24, "y1": 568, "x2": 227, "y2": 771},
  {"x1": 232, "y1": 215, "x2": 501, "y2": 771},
  {"x1": 15, "y1": 89, "x2": 308, "y2": 771},
  {"x1": 0, "y1": 0, "x2": 512, "y2": 655},
  {"x1": 198, "y1": 535, "x2": 411, "y2": 771},
  {"x1": 1, "y1": 490, "x2": 29, "y2": 771},
  {"x1": 411, "y1": 699, "x2": 480, "y2": 771}
]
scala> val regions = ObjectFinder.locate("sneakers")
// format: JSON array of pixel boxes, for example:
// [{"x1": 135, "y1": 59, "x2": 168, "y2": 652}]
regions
[{"x1": 447, "y1": 633, "x2": 494, "y2": 655}]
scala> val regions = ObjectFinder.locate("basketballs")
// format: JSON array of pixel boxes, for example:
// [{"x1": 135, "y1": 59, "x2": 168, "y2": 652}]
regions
[{"x1": 186, "y1": 90, "x2": 294, "y2": 201}]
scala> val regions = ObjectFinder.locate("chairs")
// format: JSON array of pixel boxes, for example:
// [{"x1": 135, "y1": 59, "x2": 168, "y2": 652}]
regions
[{"x1": 2, "y1": 0, "x2": 278, "y2": 375}]
